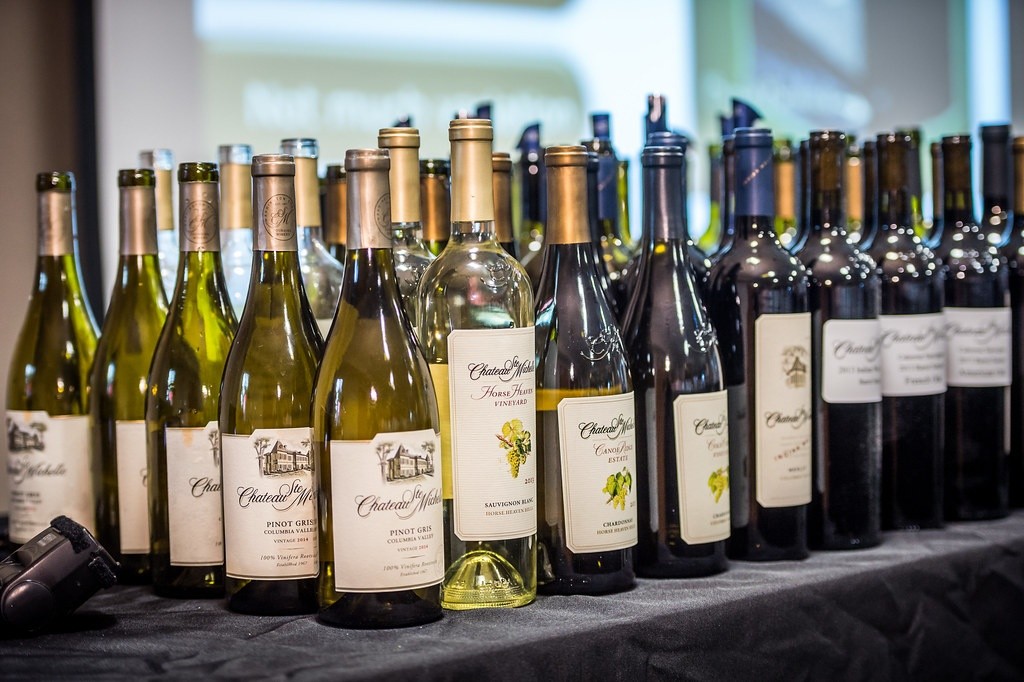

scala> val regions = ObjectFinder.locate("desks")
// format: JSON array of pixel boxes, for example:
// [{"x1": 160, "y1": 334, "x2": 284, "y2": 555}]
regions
[{"x1": 0, "y1": 509, "x2": 1024, "y2": 682}]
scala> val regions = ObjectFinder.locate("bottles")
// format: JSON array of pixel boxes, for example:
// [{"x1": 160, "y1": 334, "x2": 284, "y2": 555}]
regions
[
  {"x1": 217, "y1": 153, "x2": 326, "y2": 618},
  {"x1": 313, "y1": 161, "x2": 347, "y2": 266},
  {"x1": 217, "y1": 140, "x2": 252, "y2": 323},
  {"x1": 708, "y1": 127, "x2": 812, "y2": 562},
  {"x1": 418, "y1": 157, "x2": 450, "y2": 259},
  {"x1": 793, "y1": 127, "x2": 879, "y2": 551},
  {"x1": 139, "y1": 149, "x2": 178, "y2": 305},
  {"x1": 848, "y1": 121, "x2": 1024, "y2": 529},
  {"x1": 628, "y1": 131, "x2": 733, "y2": 577},
  {"x1": 91, "y1": 168, "x2": 171, "y2": 578},
  {"x1": 5, "y1": 172, "x2": 103, "y2": 545},
  {"x1": 144, "y1": 161, "x2": 238, "y2": 604},
  {"x1": 415, "y1": 118, "x2": 539, "y2": 610},
  {"x1": 278, "y1": 134, "x2": 344, "y2": 343},
  {"x1": 378, "y1": 128, "x2": 439, "y2": 339},
  {"x1": 491, "y1": 151, "x2": 517, "y2": 264},
  {"x1": 311, "y1": 146, "x2": 445, "y2": 629},
  {"x1": 519, "y1": 142, "x2": 641, "y2": 597}
]
